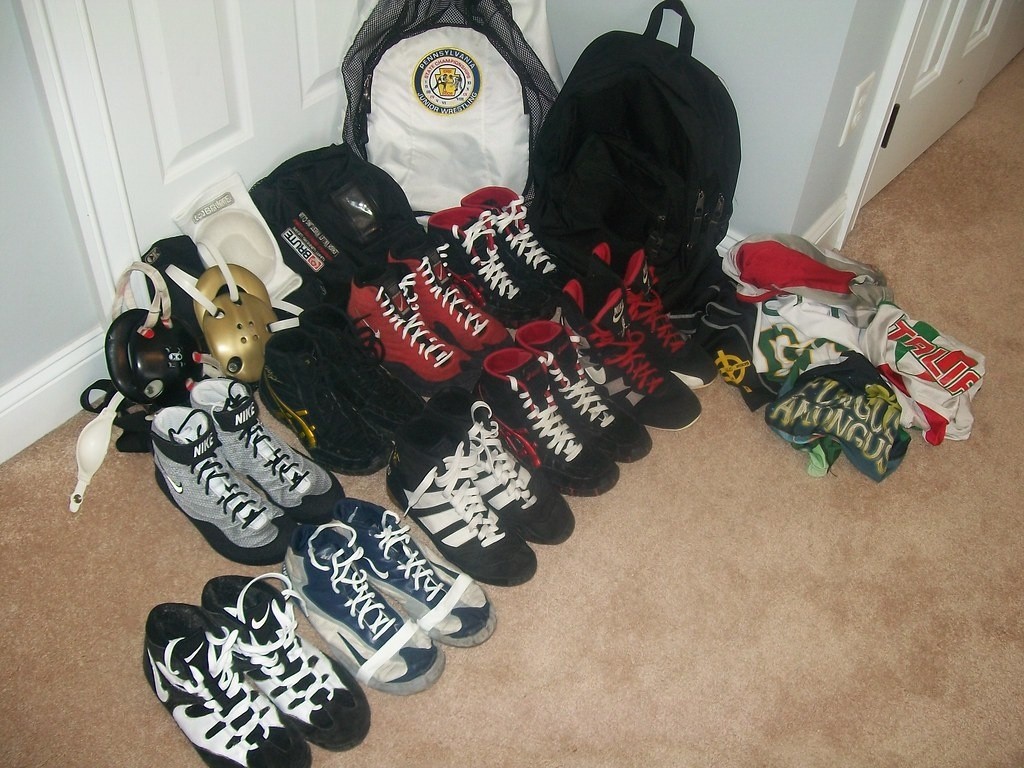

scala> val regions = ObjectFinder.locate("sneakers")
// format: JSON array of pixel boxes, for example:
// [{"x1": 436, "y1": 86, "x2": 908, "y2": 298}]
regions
[{"x1": 143, "y1": 186, "x2": 720, "y2": 768}]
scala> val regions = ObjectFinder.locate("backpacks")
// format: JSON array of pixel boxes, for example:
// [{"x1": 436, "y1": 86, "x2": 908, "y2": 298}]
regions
[
  {"x1": 529, "y1": 0, "x2": 743, "y2": 311},
  {"x1": 338, "y1": 0, "x2": 567, "y2": 231},
  {"x1": 248, "y1": 142, "x2": 429, "y2": 320}
]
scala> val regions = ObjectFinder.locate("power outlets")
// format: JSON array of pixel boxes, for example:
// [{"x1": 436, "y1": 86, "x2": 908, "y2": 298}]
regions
[{"x1": 839, "y1": 73, "x2": 877, "y2": 150}]
